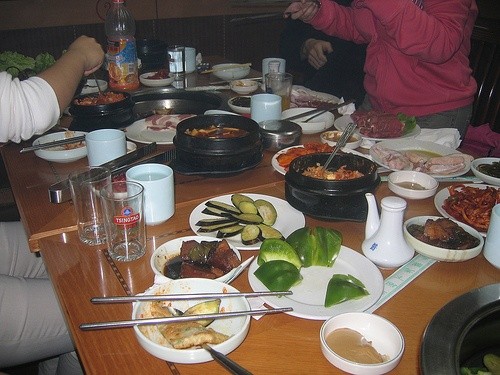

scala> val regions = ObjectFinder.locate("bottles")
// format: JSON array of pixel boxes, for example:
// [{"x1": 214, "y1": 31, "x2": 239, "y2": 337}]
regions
[{"x1": 104, "y1": 0, "x2": 140, "y2": 91}]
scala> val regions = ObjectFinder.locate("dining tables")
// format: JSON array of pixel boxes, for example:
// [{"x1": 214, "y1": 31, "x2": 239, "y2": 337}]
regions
[{"x1": 0, "y1": 54, "x2": 500, "y2": 375}]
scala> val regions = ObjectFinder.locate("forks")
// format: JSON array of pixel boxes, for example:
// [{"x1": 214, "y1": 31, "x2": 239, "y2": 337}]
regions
[{"x1": 173, "y1": 307, "x2": 255, "y2": 375}]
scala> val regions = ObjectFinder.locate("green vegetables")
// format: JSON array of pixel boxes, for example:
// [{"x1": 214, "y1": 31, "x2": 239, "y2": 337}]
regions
[
  {"x1": 0, "y1": 51, "x2": 56, "y2": 79},
  {"x1": 396, "y1": 112, "x2": 416, "y2": 134}
]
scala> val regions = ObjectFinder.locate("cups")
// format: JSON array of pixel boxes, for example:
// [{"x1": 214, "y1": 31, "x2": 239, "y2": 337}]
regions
[
  {"x1": 250, "y1": 57, "x2": 294, "y2": 124},
  {"x1": 126, "y1": 163, "x2": 175, "y2": 226},
  {"x1": 85, "y1": 129, "x2": 127, "y2": 166},
  {"x1": 167, "y1": 41, "x2": 196, "y2": 76},
  {"x1": 99, "y1": 181, "x2": 146, "y2": 262},
  {"x1": 482, "y1": 204, "x2": 500, "y2": 270},
  {"x1": 68, "y1": 166, "x2": 111, "y2": 246}
]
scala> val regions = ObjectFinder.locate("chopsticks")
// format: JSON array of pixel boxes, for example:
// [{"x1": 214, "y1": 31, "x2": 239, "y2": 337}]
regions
[
  {"x1": 20, "y1": 129, "x2": 128, "y2": 154},
  {"x1": 282, "y1": 104, "x2": 325, "y2": 121},
  {"x1": 77, "y1": 291, "x2": 294, "y2": 331},
  {"x1": 201, "y1": 62, "x2": 252, "y2": 74}
]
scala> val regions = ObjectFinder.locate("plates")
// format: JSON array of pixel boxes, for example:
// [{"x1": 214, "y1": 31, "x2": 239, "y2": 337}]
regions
[
  {"x1": 188, "y1": 193, "x2": 305, "y2": 251},
  {"x1": 271, "y1": 147, "x2": 366, "y2": 175},
  {"x1": 370, "y1": 138, "x2": 500, "y2": 262},
  {"x1": 319, "y1": 280, "x2": 500, "y2": 375},
  {"x1": 126, "y1": 96, "x2": 251, "y2": 144},
  {"x1": 150, "y1": 236, "x2": 242, "y2": 281},
  {"x1": 247, "y1": 245, "x2": 384, "y2": 321},
  {"x1": 333, "y1": 115, "x2": 421, "y2": 140}
]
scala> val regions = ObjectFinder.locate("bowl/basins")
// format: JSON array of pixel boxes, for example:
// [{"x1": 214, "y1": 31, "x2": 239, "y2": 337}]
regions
[
  {"x1": 172, "y1": 114, "x2": 263, "y2": 171},
  {"x1": 32, "y1": 131, "x2": 89, "y2": 163},
  {"x1": 126, "y1": 141, "x2": 137, "y2": 154},
  {"x1": 132, "y1": 278, "x2": 251, "y2": 364},
  {"x1": 69, "y1": 90, "x2": 135, "y2": 129},
  {"x1": 230, "y1": 80, "x2": 258, "y2": 94},
  {"x1": 212, "y1": 62, "x2": 251, "y2": 80},
  {"x1": 139, "y1": 72, "x2": 174, "y2": 87},
  {"x1": 282, "y1": 107, "x2": 362, "y2": 150},
  {"x1": 285, "y1": 152, "x2": 381, "y2": 211},
  {"x1": 387, "y1": 171, "x2": 438, "y2": 200},
  {"x1": 81, "y1": 79, "x2": 107, "y2": 95}
]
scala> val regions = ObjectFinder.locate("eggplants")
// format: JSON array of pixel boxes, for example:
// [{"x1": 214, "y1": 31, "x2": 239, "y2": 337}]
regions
[{"x1": 195, "y1": 195, "x2": 285, "y2": 246}]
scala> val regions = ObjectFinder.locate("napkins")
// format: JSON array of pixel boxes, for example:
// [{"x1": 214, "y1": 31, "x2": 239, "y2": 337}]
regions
[{"x1": 414, "y1": 127, "x2": 463, "y2": 149}]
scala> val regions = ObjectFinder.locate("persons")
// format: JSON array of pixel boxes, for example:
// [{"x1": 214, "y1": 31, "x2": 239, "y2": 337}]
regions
[
  {"x1": 282, "y1": 0, "x2": 479, "y2": 146},
  {"x1": 0, "y1": 35, "x2": 105, "y2": 375},
  {"x1": 281, "y1": 20, "x2": 367, "y2": 100}
]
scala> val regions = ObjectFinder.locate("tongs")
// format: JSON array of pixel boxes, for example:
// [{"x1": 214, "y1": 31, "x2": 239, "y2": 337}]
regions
[{"x1": 48, "y1": 142, "x2": 176, "y2": 204}]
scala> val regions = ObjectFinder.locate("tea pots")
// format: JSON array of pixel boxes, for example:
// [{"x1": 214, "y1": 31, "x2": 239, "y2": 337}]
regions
[{"x1": 361, "y1": 192, "x2": 415, "y2": 270}]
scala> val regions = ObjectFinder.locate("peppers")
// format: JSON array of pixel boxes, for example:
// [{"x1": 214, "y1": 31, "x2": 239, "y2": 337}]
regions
[{"x1": 252, "y1": 226, "x2": 369, "y2": 308}]
